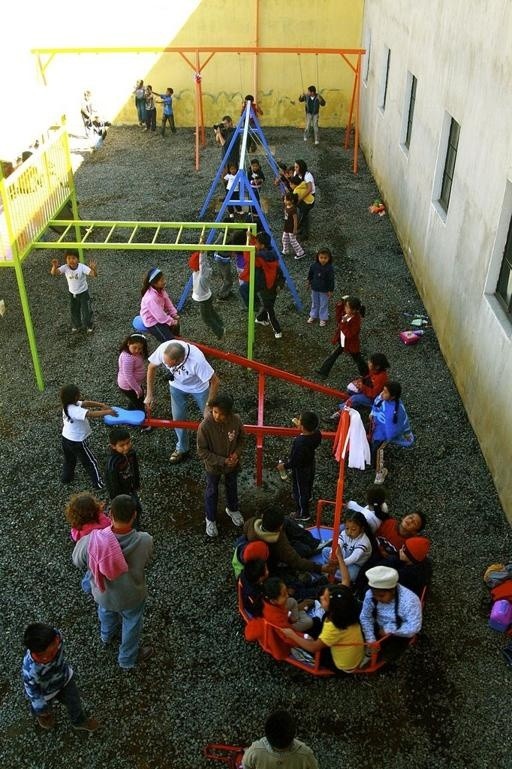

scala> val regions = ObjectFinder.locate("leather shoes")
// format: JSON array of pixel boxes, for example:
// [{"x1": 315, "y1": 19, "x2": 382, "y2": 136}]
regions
[{"x1": 136, "y1": 645, "x2": 154, "y2": 660}]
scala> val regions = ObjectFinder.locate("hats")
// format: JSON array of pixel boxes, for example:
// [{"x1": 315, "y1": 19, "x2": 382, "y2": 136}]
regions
[
  {"x1": 405, "y1": 536, "x2": 430, "y2": 563},
  {"x1": 364, "y1": 565, "x2": 400, "y2": 589}
]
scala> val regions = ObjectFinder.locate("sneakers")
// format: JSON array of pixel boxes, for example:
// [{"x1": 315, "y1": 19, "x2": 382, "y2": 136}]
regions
[
  {"x1": 72, "y1": 719, "x2": 99, "y2": 733},
  {"x1": 314, "y1": 537, "x2": 333, "y2": 553},
  {"x1": 294, "y1": 253, "x2": 306, "y2": 259},
  {"x1": 307, "y1": 318, "x2": 326, "y2": 326},
  {"x1": 169, "y1": 449, "x2": 184, "y2": 463},
  {"x1": 38, "y1": 712, "x2": 55, "y2": 729},
  {"x1": 290, "y1": 647, "x2": 315, "y2": 667},
  {"x1": 320, "y1": 412, "x2": 340, "y2": 424},
  {"x1": 374, "y1": 468, "x2": 388, "y2": 485},
  {"x1": 230, "y1": 211, "x2": 243, "y2": 218},
  {"x1": 205, "y1": 516, "x2": 219, "y2": 537},
  {"x1": 226, "y1": 507, "x2": 245, "y2": 526},
  {"x1": 289, "y1": 511, "x2": 311, "y2": 521},
  {"x1": 255, "y1": 318, "x2": 282, "y2": 338}
]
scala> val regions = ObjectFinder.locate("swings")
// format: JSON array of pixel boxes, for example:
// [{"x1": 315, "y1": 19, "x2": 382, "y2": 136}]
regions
[
  {"x1": 299, "y1": 53, "x2": 320, "y2": 119},
  {"x1": 248, "y1": 125, "x2": 314, "y2": 211},
  {"x1": 238, "y1": 52, "x2": 260, "y2": 127}
]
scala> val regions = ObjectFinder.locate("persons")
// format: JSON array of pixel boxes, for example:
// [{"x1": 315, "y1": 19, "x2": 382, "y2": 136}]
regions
[
  {"x1": 241, "y1": 708, "x2": 319, "y2": 769},
  {"x1": 20, "y1": 624, "x2": 99, "y2": 732},
  {"x1": 298, "y1": 85, "x2": 326, "y2": 144},
  {"x1": 80, "y1": 91, "x2": 111, "y2": 140},
  {"x1": 152, "y1": 87, "x2": 177, "y2": 136},
  {"x1": 144, "y1": 82, "x2": 158, "y2": 133},
  {"x1": 134, "y1": 81, "x2": 146, "y2": 126},
  {"x1": 239, "y1": 96, "x2": 263, "y2": 154}
]
